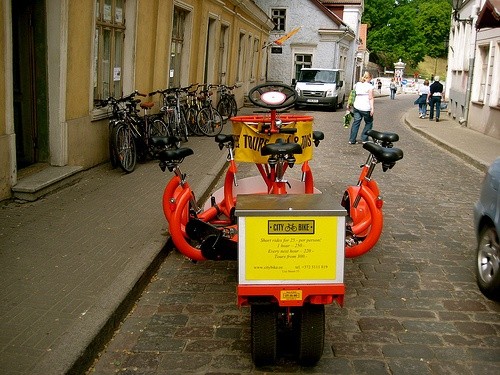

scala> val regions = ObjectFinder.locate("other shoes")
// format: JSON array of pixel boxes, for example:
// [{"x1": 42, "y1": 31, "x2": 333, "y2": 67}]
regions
[
  {"x1": 348, "y1": 141, "x2": 358, "y2": 144},
  {"x1": 422, "y1": 117, "x2": 425, "y2": 119},
  {"x1": 436, "y1": 117, "x2": 439, "y2": 122},
  {"x1": 429, "y1": 117, "x2": 433, "y2": 120},
  {"x1": 362, "y1": 141, "x2": 371, "y2": 144},
  {"x1": 419, "y1": 114, "x2": 422, "y2": 118}
]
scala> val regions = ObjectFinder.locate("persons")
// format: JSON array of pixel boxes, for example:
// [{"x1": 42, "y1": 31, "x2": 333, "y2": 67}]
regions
[
  {"x1": 417, "y1": 80, "x2": 431, "y2": 119},
  {"x1": 428, "y1": 76, "x2": 445, "y2": 122},
  {"x1": 390, "y1": 79, "x2": 396, "y2": 100},
  {"x1": 379, "y1": 79, "x2": 382, "y2": 94},
  {"x1": 347, "y1": 71, "x2": 374, "y2": 144}
]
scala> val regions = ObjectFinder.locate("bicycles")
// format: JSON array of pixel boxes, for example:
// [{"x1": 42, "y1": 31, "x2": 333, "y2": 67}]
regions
[{"x1": 92, "y1": 82, "x2": 239, "y2": 173}]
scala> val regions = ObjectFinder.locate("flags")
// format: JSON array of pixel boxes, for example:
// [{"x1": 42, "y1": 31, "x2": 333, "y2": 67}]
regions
[{"x1": 274, "y1": 28, "x2": 302, "y2": 45}]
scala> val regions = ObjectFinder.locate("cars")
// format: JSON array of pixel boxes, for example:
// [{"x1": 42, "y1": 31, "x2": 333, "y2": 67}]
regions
[{"x1": 470, "y1": 156, "x2": 500, "y2": 301}]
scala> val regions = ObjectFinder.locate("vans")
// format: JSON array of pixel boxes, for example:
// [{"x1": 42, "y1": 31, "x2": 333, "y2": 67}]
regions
[{"x1": 292, "y1": 67, "x2": 347, "y2": 112}]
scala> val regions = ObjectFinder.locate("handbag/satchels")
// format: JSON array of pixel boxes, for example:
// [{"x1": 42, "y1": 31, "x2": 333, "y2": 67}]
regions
[{"x1": 348, "y1": 87, "x2": 355, "y2": 107}]
